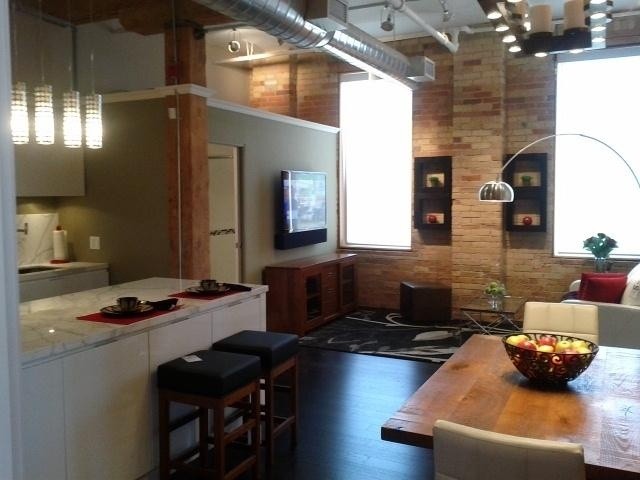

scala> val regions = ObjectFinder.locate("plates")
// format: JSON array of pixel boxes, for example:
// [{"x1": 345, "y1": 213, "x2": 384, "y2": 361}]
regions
[
  {"x1": 100, "y1": 305, "x2": 154, "y2": 316},
  {"x1": 185, "y1": 288, "x2": 230, "y2": 296}
]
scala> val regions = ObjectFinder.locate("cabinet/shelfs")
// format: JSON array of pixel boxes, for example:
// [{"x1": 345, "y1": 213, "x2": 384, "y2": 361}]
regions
[
  {"x1": 9, "y1": 6, "x2": 87, "y2": 198},
  {"x1": 264, "y1": 251, "x2": 359, "y2": 336},
  {"x1": 19, "y1": 270, "x2": 109, "y2": 302},
  {"x1": 413, "y1": 156, "x2": 451, "y2": 231},
  {"x1": 149, "y1": 296, "x2": 260, "y2": 470},
  {"x1": 22, "y1": 332, "x2": 154, "y2": 479},
  {"x1": 502, "y1": 153, "x2": 547, "y2": 234}
]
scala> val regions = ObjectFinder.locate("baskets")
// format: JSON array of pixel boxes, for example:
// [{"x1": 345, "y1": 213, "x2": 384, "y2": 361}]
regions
[{"x1": 502, "y1": 331, "x2": 600, "y2": 385}]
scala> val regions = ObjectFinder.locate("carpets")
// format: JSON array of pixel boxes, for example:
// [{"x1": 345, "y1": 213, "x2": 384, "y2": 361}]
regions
[{"x1": 299, "y1": 305, "x2": 522, "y2": 364}]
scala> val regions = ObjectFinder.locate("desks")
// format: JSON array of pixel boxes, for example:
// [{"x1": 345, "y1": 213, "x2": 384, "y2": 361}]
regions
[
  {"x1": 459, "y1": 294, "x2": 527, "y2": 345},
  {"x1": 381, "y1": 334, "x2": 640, "y2": 479}
]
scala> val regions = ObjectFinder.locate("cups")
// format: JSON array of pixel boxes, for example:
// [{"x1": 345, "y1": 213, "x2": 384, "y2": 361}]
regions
[
  {"x1": 200, "y1": 279, "x2": 218, "y2": 291},
  {"x1": 119, "y1": 296, "x2": 138, "y2": 310}
]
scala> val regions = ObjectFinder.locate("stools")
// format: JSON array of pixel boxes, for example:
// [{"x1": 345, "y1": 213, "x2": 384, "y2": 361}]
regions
[
  {"x1": 399, "y1": 281, "x2": 452, "y2": 325},
  {"x1": 212, "y1": 329, "x2": 300, "y2": 480},
  {"x1": 155, "y1": 349, "x2": 261, "y2": 479}
]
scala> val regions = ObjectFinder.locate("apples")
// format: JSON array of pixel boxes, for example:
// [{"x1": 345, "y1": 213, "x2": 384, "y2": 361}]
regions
[
  {"x1": 429, "y1": 215, "x2": 436, "y2": 223},
  {"x1": 522, "y1": 216, "x2": 532, "y2": 225},
  {"x1": 507, "y1": 335, "x2": 591, "y2": 377}
]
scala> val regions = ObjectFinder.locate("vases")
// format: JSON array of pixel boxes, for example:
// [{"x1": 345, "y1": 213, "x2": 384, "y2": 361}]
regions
[{"x1": 593, "y1": 256, "x2": 609, "y2": 273}]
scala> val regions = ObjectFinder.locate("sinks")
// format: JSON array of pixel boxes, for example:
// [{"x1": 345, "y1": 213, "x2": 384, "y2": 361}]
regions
[{"x1": 19, "y1": 266, "x2": 61, "y2": 274}]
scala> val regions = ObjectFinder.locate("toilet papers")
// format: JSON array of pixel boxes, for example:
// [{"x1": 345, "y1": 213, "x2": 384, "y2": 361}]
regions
[{"x1": 53, "y1": 229, "x2": 67, "y2": 260}]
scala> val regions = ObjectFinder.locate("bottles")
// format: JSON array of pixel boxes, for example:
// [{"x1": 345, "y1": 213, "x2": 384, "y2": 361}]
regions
[{"x1": 52, "y1": 224, "x2": 69, "y2": 261}]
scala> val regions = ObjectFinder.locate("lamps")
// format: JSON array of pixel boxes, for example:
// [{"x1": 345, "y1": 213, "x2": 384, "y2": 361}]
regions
[
  {"x1": 10, "y1": 0, "x2": 104, "y2": 149},
  {"x1": 375, "y1": 0, "x2": 613, "y2": 58},
  {"x1": 479, "y1": 132, "x2": 556, "y2": 201}
]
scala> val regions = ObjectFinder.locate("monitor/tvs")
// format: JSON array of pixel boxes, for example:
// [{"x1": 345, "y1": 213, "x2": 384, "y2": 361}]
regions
[{"x1": 281, "y1": 169, "x2": 327, "y2": 234}]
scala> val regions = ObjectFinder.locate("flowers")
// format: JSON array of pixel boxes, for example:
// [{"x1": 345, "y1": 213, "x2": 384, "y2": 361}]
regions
[{"x1": 584, "y1": 233, "x2": 617, "y2": 273}]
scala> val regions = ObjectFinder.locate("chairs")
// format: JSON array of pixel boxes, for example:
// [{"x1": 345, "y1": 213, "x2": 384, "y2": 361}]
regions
[
  {"x1": 560, "y1": 279, "x2": 640, "y2": 349},
  {"x1": 523, "y1": 300, "x2": 640, "y2": 350},
  {"x1": 432, "y1": 419, "x2": 587, "y2": 479}
]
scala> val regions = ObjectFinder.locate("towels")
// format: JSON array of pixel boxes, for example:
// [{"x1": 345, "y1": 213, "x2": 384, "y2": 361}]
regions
[
  {"x1": 168, "y1": 287, "x2": 240, "y2": 300},
  {"x1": 76, "y1": 300, "x2": 184, "y2": 325}
]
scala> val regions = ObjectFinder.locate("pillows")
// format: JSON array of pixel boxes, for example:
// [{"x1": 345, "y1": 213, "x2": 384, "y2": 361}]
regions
[
  {"x1": 577, "y1": 272, "x2": 627, "y2": 304},
  {"x1": 619, "y1": 262, "x2": 640, "y2": 306}
]
